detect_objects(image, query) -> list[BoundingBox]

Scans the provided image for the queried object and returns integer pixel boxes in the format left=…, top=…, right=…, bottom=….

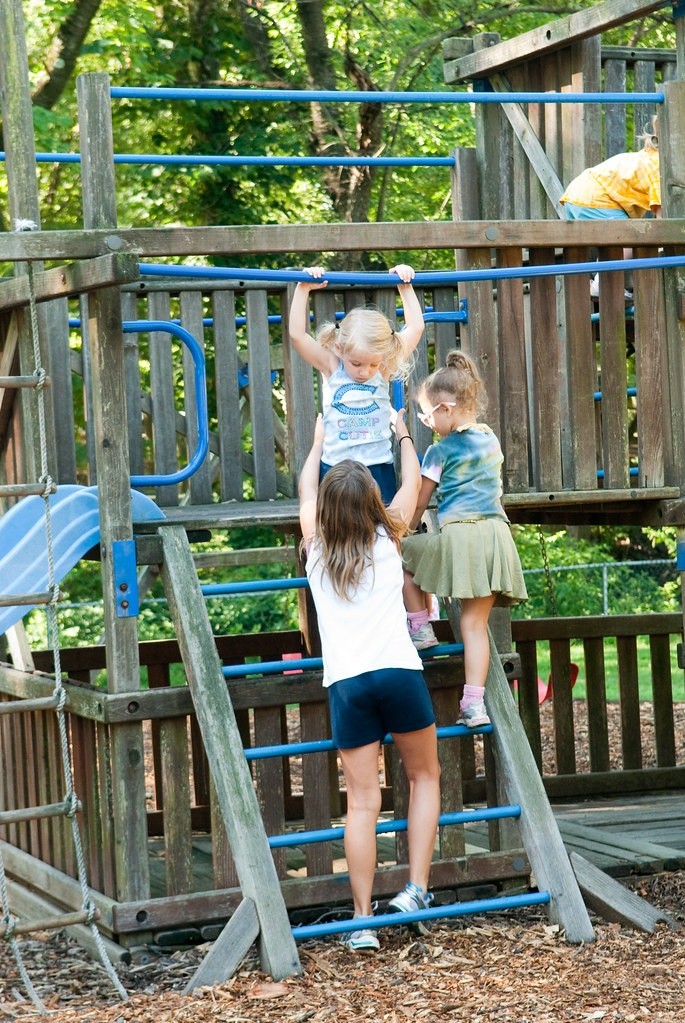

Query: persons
left=401, top=349, right=529, bottom=729
left=559, top=119, right=662, bottom=307
left=288, top=264, right=425, bottom=509
left=297, top=407, right=442, bottom=951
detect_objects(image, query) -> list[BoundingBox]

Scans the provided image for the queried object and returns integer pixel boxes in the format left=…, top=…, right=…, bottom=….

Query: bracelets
left=399, top=436, right=413, bottom=447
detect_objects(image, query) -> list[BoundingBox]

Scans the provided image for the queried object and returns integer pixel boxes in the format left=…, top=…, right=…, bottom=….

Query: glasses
left=417, top=402, right=456, bottom=427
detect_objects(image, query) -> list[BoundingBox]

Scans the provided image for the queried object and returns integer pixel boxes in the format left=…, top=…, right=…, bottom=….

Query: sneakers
left=455, top=700, right=490, bottom=727
left=409, top=623, right=439, bottom=650
left=341, top=913, right=380, bottom=953
left=388, top=882, right=432, bottom=936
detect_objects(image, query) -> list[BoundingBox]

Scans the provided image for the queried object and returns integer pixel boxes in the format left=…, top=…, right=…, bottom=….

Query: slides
left=0, top=482, right=169, bottom=632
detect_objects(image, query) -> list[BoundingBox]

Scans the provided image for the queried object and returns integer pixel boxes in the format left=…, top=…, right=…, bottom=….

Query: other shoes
left=590, top=274, right=633, bottom=298
